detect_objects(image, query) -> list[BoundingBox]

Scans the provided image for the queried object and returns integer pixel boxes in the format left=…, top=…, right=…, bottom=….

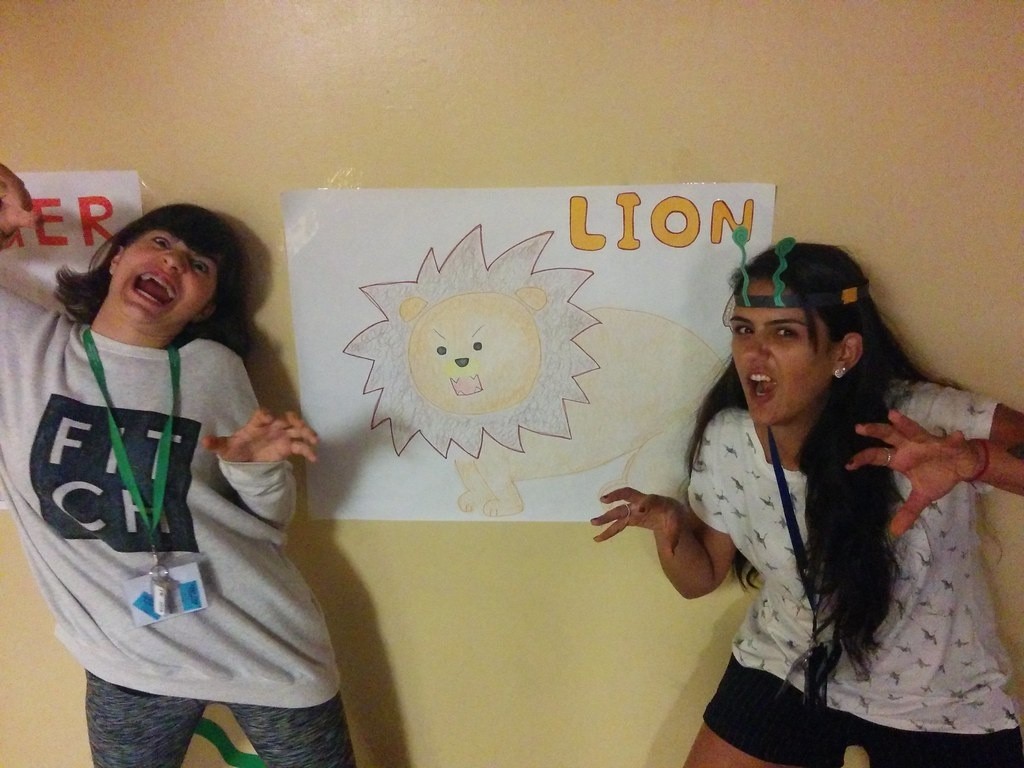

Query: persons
left=590, top=241, right=1023, bottom=768
left=0, top=162, right=355, bottom=768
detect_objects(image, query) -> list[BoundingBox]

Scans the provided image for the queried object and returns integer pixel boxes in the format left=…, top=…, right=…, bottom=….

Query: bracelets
left=964, top=439, right=989, bottom=482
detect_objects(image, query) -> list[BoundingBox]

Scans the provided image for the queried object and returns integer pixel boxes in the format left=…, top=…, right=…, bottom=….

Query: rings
left=625, top=504, right=630, bottom=516
left=884, top=447, right=891, bottom=464
